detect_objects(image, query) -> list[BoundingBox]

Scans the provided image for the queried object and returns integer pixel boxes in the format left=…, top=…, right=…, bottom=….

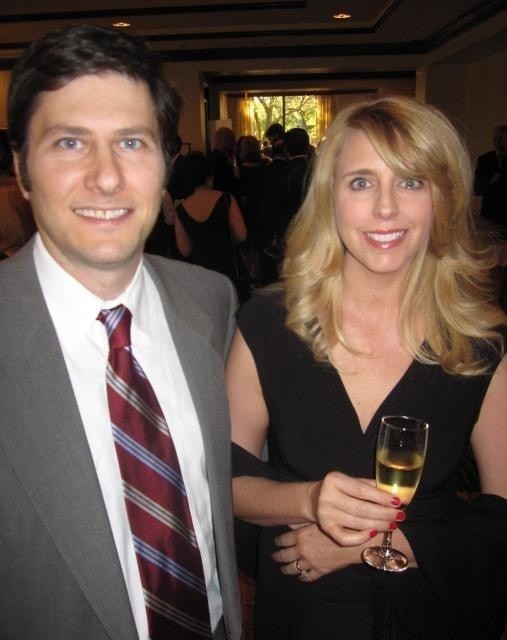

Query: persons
left=146, top=124, right=336, bottom=304
left=221, top=96, right=506, bottom=638
left=1, top=27, right=241, bottom=639
left=473, top=124, right=506, bottom=308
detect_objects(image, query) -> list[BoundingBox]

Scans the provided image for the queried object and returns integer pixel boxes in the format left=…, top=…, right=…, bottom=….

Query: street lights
left=232, top=253, right=248, bottom=280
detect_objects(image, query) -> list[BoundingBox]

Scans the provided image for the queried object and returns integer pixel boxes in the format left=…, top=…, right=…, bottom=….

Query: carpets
left=97, top=305, right=212, bottom=639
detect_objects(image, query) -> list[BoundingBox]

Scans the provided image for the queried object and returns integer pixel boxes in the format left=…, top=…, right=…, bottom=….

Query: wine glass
left=296, top=560, right=302, bottom=573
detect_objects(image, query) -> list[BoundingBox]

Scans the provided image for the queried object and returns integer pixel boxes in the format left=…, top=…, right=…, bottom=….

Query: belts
left=361, top=414, right=431, bottom=572
